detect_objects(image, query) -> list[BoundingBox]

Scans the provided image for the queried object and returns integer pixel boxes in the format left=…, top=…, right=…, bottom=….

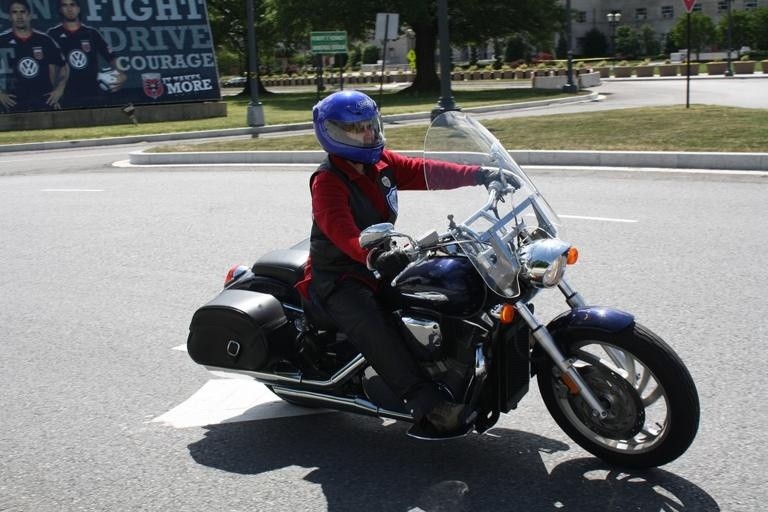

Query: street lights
left=400, top=21, right=416, bottom=49
left=606, top=12, right=621, bottom=57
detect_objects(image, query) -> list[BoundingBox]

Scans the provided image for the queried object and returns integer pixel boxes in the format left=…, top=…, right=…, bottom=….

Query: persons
left=294, top=88, right=523, bottom=434
left=46, top=0, right=127, bottom=110
left=1, top=0, right=70, bottom=111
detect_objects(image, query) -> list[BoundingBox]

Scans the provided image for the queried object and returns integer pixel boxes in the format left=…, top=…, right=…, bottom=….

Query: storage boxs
left=184, top=287, right=291, bottom=371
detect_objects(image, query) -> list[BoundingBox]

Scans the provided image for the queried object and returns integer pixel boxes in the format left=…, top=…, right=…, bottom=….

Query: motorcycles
left=182, top=109, right=700, bottom=472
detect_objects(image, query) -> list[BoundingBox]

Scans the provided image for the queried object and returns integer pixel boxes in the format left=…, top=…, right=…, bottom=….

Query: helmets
left=314, top=91, right=385, bottom=165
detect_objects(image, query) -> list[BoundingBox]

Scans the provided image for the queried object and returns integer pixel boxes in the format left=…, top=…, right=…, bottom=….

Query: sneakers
left=427, top=402, right=477, bottom=428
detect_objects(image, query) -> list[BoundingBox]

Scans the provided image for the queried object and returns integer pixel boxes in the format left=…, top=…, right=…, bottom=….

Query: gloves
left=369, top=250, right=406, bottom=278
left=477, top=168, right=521, bottom=190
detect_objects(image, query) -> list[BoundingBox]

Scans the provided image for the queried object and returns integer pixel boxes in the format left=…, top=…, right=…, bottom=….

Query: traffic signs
left=309, top=30, right=348, bottom=55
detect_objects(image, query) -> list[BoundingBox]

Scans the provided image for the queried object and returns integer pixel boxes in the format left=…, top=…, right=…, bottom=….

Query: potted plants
left=591, top=60, right=610, bottom=78
left=658, top=61, right=678, bottom=76
left=516, top=64, right=532, bottom=79
left=264, top=71, right=392, bottom=88
left=759, top=59, right=768, bottom=73
left=465, top=65, right=480, bottom=80
left=453, top=66, right=464, bottom=80
left=636, top=58, right=655, bottom=77
left=534, top=63, right=550, bottom=77
left=500, top=65, right=515, bottom=80
left=494, top=70, right=502, bottom=80
left=680, top=60, right=699, bottom=74
left=706, top=58, right=729, bottom=75
left=732, top=55, right=757, bottom=75
left=392, top=70, right=407, bottom=82
left=483, top=66, right=492, bottom=79
left=408, top=68, right=416, bottom=82
left=552, top=61, right=567, bottom=76
left=613, top=60, right=634, bottom=78
left=574, top=61, right=589, bottom=73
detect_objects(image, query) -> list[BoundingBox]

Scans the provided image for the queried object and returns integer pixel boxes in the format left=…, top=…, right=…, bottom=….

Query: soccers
left=98, top=67, right=119, bottom=92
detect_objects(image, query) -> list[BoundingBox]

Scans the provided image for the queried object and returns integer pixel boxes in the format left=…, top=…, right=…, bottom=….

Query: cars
left=223, top=77, right=248, bottom=88
